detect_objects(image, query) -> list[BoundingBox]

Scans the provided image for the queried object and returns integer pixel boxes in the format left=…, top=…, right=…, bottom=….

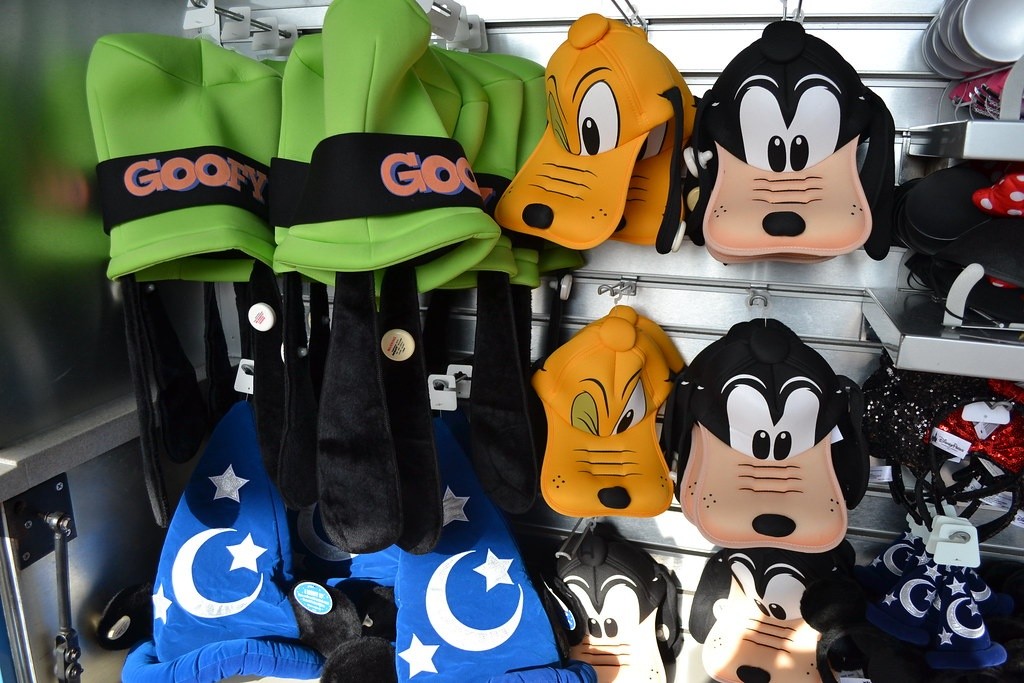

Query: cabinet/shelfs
left=0, top=0, right=1024, bottom=683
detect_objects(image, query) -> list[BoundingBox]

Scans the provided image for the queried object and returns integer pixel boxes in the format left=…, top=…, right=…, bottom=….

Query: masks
left=494, top=13, right=691, bottom=250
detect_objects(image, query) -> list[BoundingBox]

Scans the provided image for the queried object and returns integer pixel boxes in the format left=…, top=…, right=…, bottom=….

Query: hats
left=269, top=0, right=587, bottom=555
left=530, top=307, right=686, bottom=517
left=525, top=521, right=680, bottom=683
left=396, top=422, right=597, bottom=683
left=682, top=21, right=895, bottom=264
left=84, top=34, right=329, bottom=525
left=676, top=318, right=868, bottom=553
left=119, top=400, right=323, bottom=683
left=603, top=25, right=697, bottom=255
left=688, top=538, right=856, bottom=682
